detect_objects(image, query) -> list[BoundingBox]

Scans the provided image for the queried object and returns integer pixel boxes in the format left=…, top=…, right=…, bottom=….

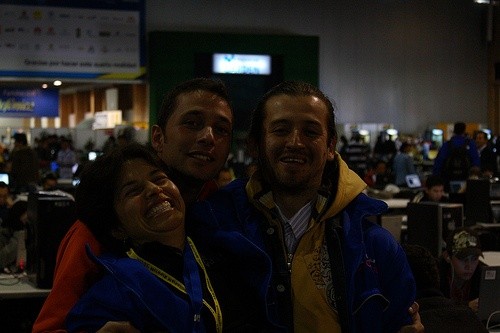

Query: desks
left=0, top=272, right=52, bottom=300
left=358, top=158, right=500, bottom=332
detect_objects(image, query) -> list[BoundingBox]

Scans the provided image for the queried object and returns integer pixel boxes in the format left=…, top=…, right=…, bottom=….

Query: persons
left=0, top=76, right=500, bottom=333
left=63, top=149, right=260, bottom=333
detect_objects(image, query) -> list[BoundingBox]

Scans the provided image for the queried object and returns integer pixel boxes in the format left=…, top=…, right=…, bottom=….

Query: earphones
left=446, top=256, right=450, bottom=262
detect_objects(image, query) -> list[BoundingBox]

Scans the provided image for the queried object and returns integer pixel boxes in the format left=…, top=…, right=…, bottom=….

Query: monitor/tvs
left=476, top=266, right=500, bottom=323
left=404, top=174, right=422, bottom=188
left=0, top=173, right=10, bottom=186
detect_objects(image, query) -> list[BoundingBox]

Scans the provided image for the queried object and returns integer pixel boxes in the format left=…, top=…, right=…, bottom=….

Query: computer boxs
left=407, top=201, right=464, bottom=259
left=25, top=190, right=76, bottom=290
left=448, top=179, right=492, bottom=226
